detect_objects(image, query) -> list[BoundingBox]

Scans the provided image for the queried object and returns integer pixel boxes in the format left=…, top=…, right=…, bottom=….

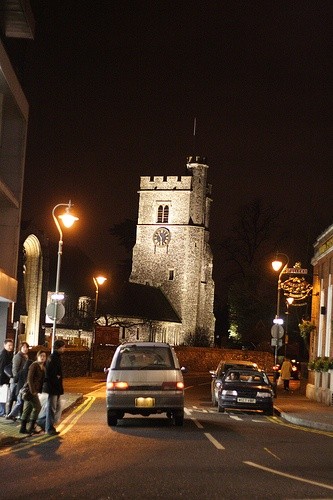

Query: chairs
left=123, top=354, right=136, bottom=365
left=230, top=371, right=240, bottom=380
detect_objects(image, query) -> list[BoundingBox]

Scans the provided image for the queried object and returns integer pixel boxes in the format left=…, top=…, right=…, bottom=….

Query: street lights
left=271, top=252, right=289, bottom=382
left=88, top=272, right=108, bottom=377
left=50, top=200, right=79, bottom=355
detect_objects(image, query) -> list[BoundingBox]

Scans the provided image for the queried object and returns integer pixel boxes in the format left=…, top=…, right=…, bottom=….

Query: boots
left=20, top=421, right=28, bottom=433
left=29, top=421, right=39, bottom=434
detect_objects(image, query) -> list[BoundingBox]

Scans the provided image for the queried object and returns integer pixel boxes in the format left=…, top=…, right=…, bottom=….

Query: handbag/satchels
left=19, top=383, right=34, bottom=401
left=3, top=364, right=14, bottom=377
left=0, top=384, right=12, bottom=403
left=277, top=372, right=283, bottom=386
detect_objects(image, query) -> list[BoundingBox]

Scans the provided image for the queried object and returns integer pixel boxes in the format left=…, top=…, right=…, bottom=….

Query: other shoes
left=37, top=423, right=45, bottom=432
left=5, top=411, right=18, bottom=419
left=11, top=416, right=17, bottom=420
left=11, top=415, right=17, bottom=420
left=46, top=428, right=60, bottom=436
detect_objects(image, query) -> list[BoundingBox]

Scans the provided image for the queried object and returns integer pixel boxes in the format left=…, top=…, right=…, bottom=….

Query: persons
left=20, top=349, right=60, bottom=433
left=281, top=357, right=293, bottom=390
left=0, top=337, right=37, bottom=421
left=227, top=372, right=241, bottom=382
left=43, top=340, right=66, bottom=434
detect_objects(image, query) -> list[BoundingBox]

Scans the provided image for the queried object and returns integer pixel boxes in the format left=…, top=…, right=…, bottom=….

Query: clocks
left=152, top=226, right=172, bottom=248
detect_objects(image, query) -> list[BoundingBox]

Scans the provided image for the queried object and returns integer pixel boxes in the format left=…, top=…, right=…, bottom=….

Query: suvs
left=210, top=359, right=259, bottom=406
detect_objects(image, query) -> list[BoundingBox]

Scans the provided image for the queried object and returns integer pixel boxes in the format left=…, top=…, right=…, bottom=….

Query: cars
left=214, top=368, right=275, bottom=416
left=275, top=360, right=299, bottom=380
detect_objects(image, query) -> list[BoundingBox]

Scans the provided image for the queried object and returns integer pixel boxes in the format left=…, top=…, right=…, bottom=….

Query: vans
left=103, top=341, right=186, bottom=426
